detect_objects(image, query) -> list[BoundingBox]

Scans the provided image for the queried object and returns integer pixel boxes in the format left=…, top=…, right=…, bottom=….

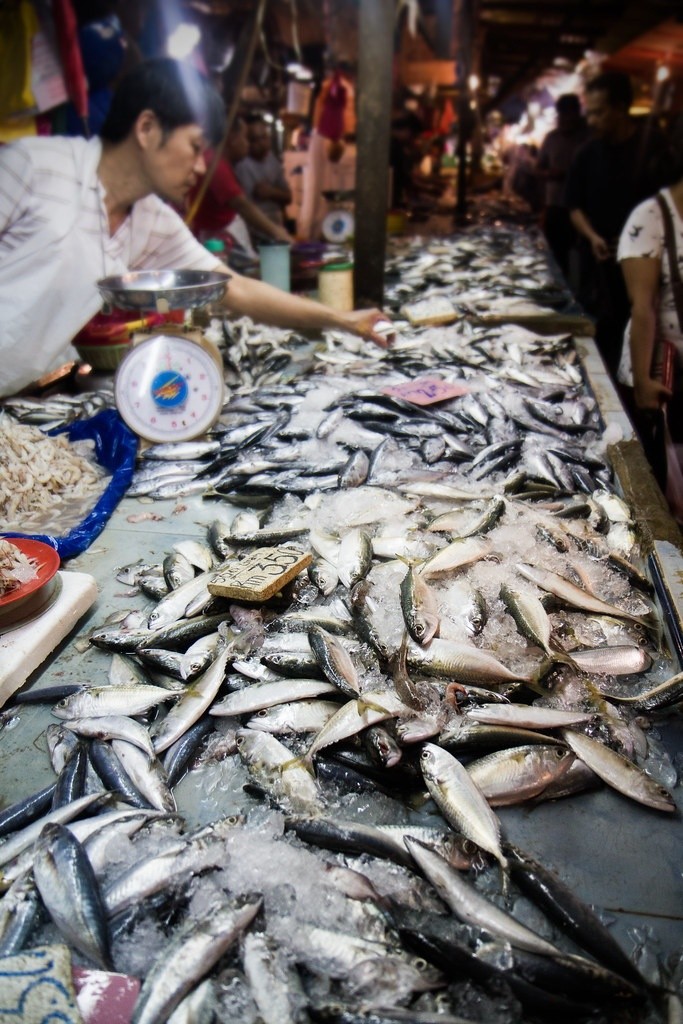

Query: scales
left=320, top=188, right=355, bottom=244
left=92, top=268, right=231, bottom=452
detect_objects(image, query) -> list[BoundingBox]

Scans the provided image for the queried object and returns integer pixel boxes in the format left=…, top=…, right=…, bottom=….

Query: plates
left=0, top=536, right=61, bottom=610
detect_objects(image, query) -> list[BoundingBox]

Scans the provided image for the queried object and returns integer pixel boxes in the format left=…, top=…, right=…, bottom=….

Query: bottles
left=203, top=236, right=228, bottom=267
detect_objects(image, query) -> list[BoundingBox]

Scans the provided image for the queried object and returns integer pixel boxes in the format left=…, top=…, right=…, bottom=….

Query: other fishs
left=5, top=389, right=117, bottom=433
left=122, top=223, right=616, bottom=500
left=0, top=488, right=683, bottom=1024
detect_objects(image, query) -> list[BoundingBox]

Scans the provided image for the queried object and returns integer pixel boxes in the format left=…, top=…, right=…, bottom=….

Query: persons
left=390, top=101, right=425, bottom=211
left=504, top=69, right=664, bottom=370
left=616, top=114, right=683, bottom=492
left=0, top=59, right=395, bottom=400
left=192, top=114, right=296, bottom=251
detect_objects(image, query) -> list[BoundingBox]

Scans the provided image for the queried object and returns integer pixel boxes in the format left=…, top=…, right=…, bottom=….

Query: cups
left=317, top=263, right=354, bottom=313
left=257, top=240, right=291, bottom=295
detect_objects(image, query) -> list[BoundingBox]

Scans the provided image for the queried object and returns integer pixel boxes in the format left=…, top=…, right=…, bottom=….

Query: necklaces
left=97, top=178, right=133, bottom=315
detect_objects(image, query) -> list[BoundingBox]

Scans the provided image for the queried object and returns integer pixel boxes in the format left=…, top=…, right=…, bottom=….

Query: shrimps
left=0, top=410, right=114, bottom=599
